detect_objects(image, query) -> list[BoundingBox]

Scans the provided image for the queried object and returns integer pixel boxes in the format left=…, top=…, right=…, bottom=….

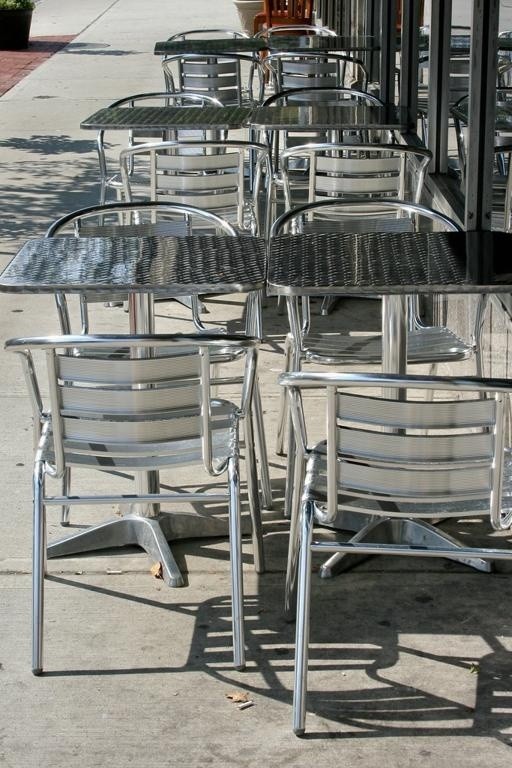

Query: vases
left=235, top=2, right=265, bottom=38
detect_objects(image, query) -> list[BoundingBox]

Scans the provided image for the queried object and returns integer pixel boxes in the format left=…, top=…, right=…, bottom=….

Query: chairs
left=277, top=370, right=512, bottom=738
left=254, top=24, right=336, bottom=60
left=5, top=334, right=263, bottom=675
left=253, top=52, right=370, bottom=95
left=416, top=48, right=473, bottom=144
left=118, top=140, right=273, bottom=228
left=96, top=91, right=228, bottom=226
left=252, top=0, right=313, bottom=83
left=46, top=199, right=241, bottom=334
left=280, top=142, right=434, bottom=231
left=159, top=51, right=267, bottom=194
left=164, top=28, right=258, bottom=102
left=257, top=87, right=384, bottom=240
left=271, top=198, right=465, bottom=375
left=451, top=62, right=511, bottom=198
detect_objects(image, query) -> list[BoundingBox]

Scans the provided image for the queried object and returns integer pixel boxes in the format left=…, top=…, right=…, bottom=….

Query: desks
left=397, top=34, right=512, bottom=53
left=269, top=34, right=378, bottom=60
left=267, top=231, right=512, bottom=580
left=242, top=102, right=403, bottom=318
left=0, top=236, right=267, bottom=589
left=81, top=108, right=246, bottom=314
left=153, top=40, right=267, bottom=57
left=449, top=103, right=511, bottom=132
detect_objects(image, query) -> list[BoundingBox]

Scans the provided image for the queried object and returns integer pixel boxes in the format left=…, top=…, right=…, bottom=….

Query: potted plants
left=0, top=0, right=35, bottom=52
